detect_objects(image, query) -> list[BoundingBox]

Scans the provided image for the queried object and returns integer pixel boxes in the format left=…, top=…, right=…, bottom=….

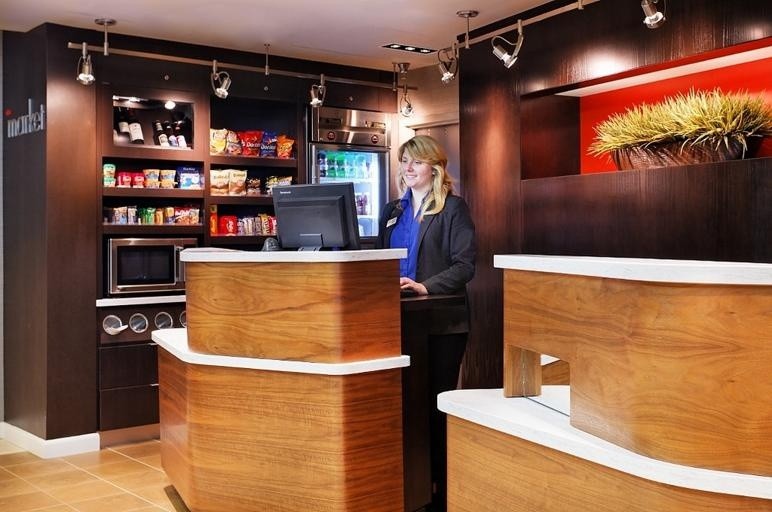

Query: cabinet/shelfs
left=96, top=80, right=307, bottom=447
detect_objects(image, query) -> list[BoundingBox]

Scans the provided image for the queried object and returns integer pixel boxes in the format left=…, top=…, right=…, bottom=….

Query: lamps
left=437, top=0, right=667, bottom=85
left=161, top=99, right=176, bottom=110
left=67, top=41, right=419, bottom=117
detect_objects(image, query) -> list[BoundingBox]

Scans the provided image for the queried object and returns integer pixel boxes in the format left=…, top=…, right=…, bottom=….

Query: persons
left=370, top=135, right=479, bottom=511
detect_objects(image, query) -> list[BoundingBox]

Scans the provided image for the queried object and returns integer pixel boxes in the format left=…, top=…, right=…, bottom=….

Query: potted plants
left=586, top=83, right=772, bottom=171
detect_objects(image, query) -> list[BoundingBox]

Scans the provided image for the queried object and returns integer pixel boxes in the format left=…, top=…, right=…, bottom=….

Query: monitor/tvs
left=270, top=181, right=363, bottom=251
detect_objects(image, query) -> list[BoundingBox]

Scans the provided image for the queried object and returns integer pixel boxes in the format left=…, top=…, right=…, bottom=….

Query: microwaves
left=106, top=238, right=197, bottom=295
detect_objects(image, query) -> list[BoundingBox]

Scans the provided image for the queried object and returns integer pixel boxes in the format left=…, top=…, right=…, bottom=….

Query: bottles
left=114, top=106, right=188, bottom=150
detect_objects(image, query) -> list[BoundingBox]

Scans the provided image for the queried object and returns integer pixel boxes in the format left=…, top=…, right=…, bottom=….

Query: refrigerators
left=306, top=107, right=390, bottom=250
